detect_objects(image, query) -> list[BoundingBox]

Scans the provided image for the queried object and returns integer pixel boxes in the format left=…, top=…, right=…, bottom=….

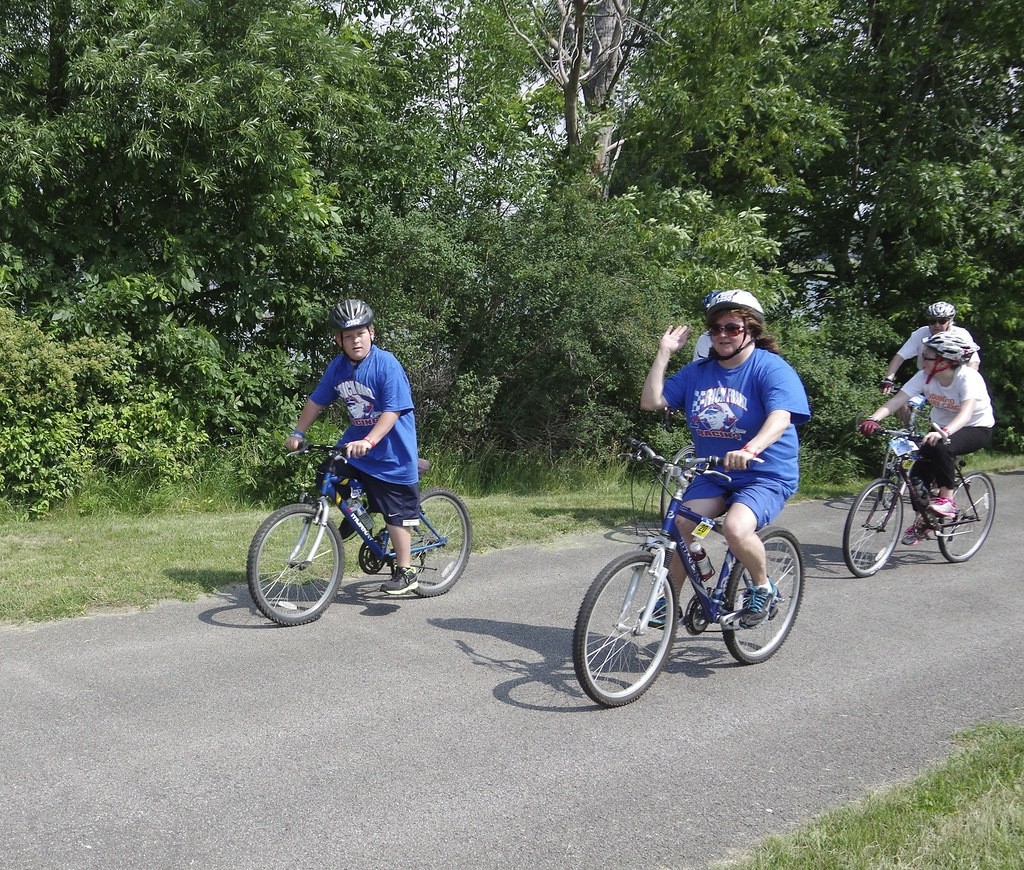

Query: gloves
left=860, top=420, right=881, bottom=436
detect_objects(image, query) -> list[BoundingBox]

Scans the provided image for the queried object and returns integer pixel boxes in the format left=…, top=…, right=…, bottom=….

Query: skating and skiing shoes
left=739, top=577, right=779, bottom=628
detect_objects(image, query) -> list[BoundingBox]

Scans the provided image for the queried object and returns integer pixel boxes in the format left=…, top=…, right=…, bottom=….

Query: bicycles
left=883, top=377, right=933, bottom=509
left=842, top=424, right=996, bottom=577
left=571, top=437, right=806, bottom=707
left=246, top=442, right=473, bottom=626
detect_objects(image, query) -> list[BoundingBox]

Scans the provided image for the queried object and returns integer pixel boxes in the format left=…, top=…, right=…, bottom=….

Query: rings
left=730, top=460, right=735, bottom=463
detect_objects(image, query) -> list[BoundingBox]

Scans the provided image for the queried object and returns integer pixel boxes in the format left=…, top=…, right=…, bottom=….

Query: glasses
left=710, top=323, right=750, bottom=337
left=922, top=353, right=937, bottom=361
left=928, top=318, right=951, bottom=324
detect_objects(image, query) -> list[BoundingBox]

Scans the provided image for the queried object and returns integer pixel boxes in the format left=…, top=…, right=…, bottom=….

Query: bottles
left=347, top=498, right=375, bottom=530
left=911, top=476, right=930, bottom=506
left=930, top=483, right=940, bottom=497
left=689, top=542, right=712, bottom=579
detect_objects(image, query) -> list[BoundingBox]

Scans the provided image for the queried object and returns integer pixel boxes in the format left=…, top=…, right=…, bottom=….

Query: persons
left=879, top=302, right=980, bottom=498
left=692, top=291, right=713, bottom=362
left=284, top=300, right=422, bottom=594
left=857, top=333, right=995, bottom=546
left=640, top=290, right=811, bottom=627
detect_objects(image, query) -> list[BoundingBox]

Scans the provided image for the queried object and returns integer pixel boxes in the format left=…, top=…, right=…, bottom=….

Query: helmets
left=927, top=301, right=956, bottom=318
left=703, top=289, right=764, bottom=323
left=921, top=332, right=981, bottom=360
left=331, top=298, right=374, bottom=329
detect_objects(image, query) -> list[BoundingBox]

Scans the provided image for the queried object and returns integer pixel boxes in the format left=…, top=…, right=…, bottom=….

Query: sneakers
left=380, top=565, right=419, bottom=595
left=335, top=498, right=374, bottom=542
left=927, top=497, right=956, bottom=518
left=639, top=597, right=683, bottom=630
left=901, top=524, right=932, bottom=546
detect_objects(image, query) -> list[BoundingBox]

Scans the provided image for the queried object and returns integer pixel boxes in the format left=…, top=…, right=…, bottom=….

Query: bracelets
left=291, top=431, right=306, bottom=439
left=364, top=437, right=376, bottom=447
left=942, top=427, right=952, bottom=436
left=743, top=446, right=758, bottom=457
